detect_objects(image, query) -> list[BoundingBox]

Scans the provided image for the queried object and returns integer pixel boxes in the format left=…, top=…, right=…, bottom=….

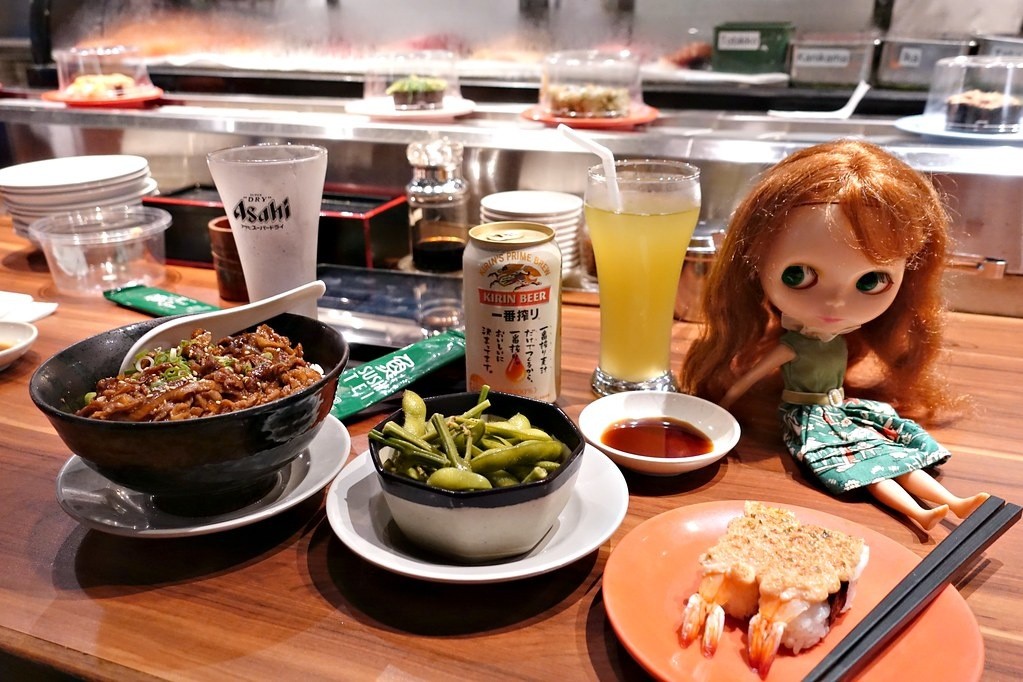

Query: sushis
left=679, top=503, right=871, bottom=674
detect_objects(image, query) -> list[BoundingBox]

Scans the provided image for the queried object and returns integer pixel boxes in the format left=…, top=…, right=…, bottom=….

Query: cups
left=208, top=215, right=250, bottom=304
left=206, top=142, right=328, bottom=320
left=586, top=158, right=703, bottom=399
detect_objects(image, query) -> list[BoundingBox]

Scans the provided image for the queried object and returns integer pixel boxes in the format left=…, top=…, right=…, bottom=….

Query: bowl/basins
left=27, top=310, right=348, bottom=518
left=368, top=391, right=585, bottom=561
left=1, top=154, right=174, bottom=295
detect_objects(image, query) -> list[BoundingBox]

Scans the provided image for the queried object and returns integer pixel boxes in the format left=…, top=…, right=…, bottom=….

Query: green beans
left=369, top=383, right=571, bottom=492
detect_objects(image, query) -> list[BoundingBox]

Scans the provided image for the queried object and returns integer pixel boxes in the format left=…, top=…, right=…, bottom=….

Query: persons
left=681, top=137, right=992, bottom=533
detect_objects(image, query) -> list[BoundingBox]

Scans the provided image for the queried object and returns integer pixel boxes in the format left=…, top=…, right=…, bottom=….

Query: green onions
left=84, top=339, right=273, bottom=405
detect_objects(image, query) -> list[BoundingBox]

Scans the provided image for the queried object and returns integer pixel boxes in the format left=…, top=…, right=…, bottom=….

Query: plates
left=603, top=499, right=987, bottom=682
left=326, top=441, right=633, bottom=584
left=0, top=320, right=38, bottom=371
left=520, top=103, right=659, bottom=134
left=57, top=414, right=352, bottom=538
left=894, top=112, right=1023, bottom=145
left=40, top=85, right=164, bottom=108
left=480, top=190, right=585, bottom=281
left=577, top=389, right=741, bottom=477
left=344, top=96, right=475, bottom=122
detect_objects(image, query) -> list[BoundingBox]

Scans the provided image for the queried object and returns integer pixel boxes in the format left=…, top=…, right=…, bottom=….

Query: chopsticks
left=800, top=494, right=1023, bottom=682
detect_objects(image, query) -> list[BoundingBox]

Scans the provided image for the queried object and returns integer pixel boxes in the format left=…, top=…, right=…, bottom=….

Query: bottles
left=674, top=219, right=733, bottom=324
left=407, top=139, right=471, bottom=273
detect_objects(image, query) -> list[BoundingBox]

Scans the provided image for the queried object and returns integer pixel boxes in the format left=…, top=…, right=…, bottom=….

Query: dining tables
left=0, top=206, right=1023, bottom=681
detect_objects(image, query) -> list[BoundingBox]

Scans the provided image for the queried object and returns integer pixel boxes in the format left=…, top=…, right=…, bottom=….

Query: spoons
left=118, top=280, right=326, bottom=377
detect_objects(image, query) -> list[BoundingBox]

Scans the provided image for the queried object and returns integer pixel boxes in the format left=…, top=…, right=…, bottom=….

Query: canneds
left=461, top=219, right=564, bottom=404
